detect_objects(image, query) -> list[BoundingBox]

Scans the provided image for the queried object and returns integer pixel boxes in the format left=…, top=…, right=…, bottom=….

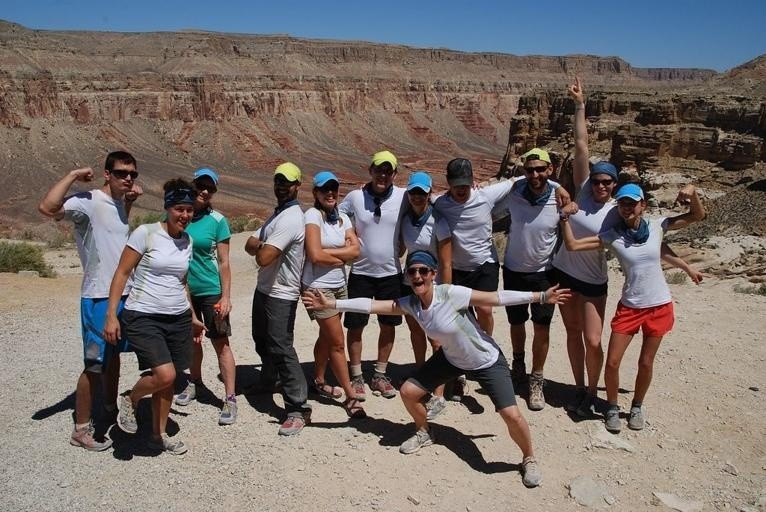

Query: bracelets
left=124, top=192, right=137, bottom=202
left=573, top=103, right=584, bottom=110
left=256, top=242, right=263, bottom=249
left=559, top=213, right=570, bottom=223
left=538, top=291, right=546, bottom=305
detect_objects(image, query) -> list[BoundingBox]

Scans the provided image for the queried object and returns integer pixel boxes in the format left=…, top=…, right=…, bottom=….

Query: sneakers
left=521, top=456, right=539, bottom=488
left=399, top=425, right=435, bottom=454
left=69, top=376, right=238, bottom=455
left=242, top=368, right=398, bottom=436
left=566, top=387, right=644, bottom=431
left=425, top=359, right=545, bottom=420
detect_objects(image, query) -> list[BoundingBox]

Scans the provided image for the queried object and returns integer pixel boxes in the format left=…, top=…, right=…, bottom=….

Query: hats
left=616, top=183, right=644, bottom=201
left=521, top=148, right=552, bottom=164
left=313, top=170, right=339, bottom=188
left=407, top=172, right=432, bottom=194
left=274, top=161, right=302, bottom=183
left=193, top=168, right=218, bottom=185
left=590, top=161, right=618, bottom=182
left=371, top=150, right=398, bottom=171
left=447, top=158, right=472, bottom=187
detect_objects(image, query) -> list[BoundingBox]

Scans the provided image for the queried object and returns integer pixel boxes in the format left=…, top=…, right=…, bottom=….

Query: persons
left=397, top=171, right=453, bottom=420
left=302, top=249, right=573, bottom=486
left=336, top=151, right=484, bottom=402
left=160, top=168, right=237, bottom=426
left=102, top=179, right=209, bottom=456
left=303, top=170, right=366, bottom=420
left=244, top=161, right=314, bottom=437
left=491, top=147, right=564, bottom=412
left=551, top=77, right=712, bottom=417
left=38, top=150, right=144, bottom=451
left=359, top=157, right=573, bottom=403
left=558, top=182, right=704, bottom=433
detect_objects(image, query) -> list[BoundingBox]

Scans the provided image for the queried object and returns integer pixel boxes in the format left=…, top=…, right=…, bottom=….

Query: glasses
left=174, top=189, right=198, bottom=200
left=407, top=267, right=432, bottom=275
left=374, top=197, right=381, bottom=216
left=592, top=179, right=612, bottom=185
left=524, top=166, right=549, bottom=172
left=111, top=170, right=138, bottom=179
left=195, top=183, right=217, bottom=193
